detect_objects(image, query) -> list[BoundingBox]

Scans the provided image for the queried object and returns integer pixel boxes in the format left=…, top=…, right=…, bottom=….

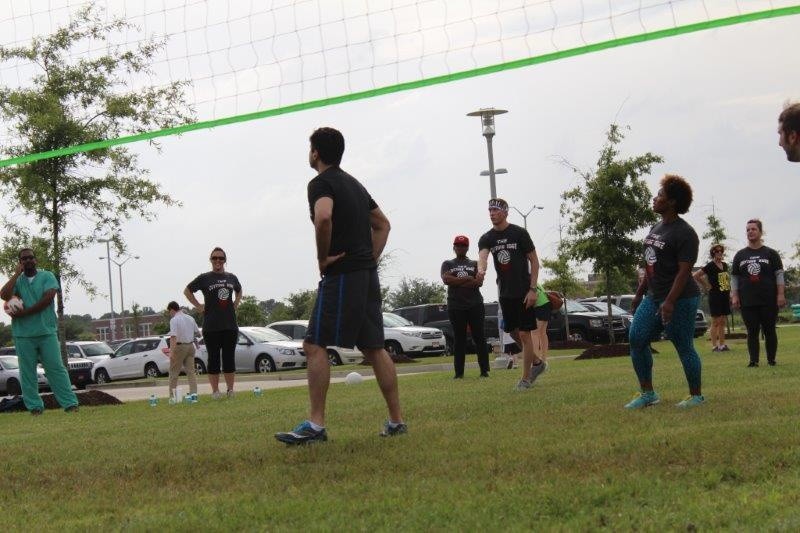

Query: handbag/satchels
left=546, top=291, right=563, bottom=310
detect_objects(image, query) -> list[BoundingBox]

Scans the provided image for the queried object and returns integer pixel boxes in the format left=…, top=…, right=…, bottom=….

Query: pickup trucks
left=391, top=303, right=500, bottom=354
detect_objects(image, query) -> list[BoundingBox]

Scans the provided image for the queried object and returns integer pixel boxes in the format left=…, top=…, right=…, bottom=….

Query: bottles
left=191, top=392, right=198, bottom=403
left=185, top=392, right=191, bottom=403
left=149, top=395, right=157, bottom=407
left=254, top=387, right=259, bottom=395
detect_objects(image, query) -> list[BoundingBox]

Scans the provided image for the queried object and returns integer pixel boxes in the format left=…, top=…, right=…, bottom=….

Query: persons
left=275, top=127, right=407, bottom=444
left=0, top=248, right=79, bottom=414
left=439, top=198, right=552, bottom=389
left=692, top=244, right=734, bottom=352
left=167, top=302, right=201, bottom=399
left=625, top=174, right=707, bottom=410
left=184, top=248, right=242, bottom=398
left=730, top=220, right=784, bottom=367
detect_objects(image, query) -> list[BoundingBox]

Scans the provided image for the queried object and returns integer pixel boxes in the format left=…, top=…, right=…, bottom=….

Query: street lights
left=509, top=205, right=544, bottom=230
left=465, top=104, right=509, bottom=199
left=98, top=239, right=119, bottom=340
left=99, top=254, right=142, bottom=338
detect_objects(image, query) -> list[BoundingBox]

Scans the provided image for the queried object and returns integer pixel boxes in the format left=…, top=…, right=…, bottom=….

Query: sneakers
left=676, top=394, right=704, bottom=405
left=380, top=422, right=408, bottom=436
left=274, top=421, right=328, bottom=444
left=626, top=391, right=660, bottom=408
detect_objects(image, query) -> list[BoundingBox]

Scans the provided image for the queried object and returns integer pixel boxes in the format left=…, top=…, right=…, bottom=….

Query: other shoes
left=213, top=390, right=221, bottom=399
left=31, top=407, right=43, bottom=415
left=713, top=345, right=730, bottom=351
left=227, top=389, right=235, bottom=397
left=64, top=406, right=78, bottom=413
left=767, top=360, right=776, bottom=367
left=515, top=360, right=546, bottom=389
left=748, top=362, right=758, bottom=367
left=507, top=358, right=518, bottom=369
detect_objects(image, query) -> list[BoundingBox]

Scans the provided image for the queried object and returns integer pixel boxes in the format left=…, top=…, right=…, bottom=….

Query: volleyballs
left=345, top=371, right=364, bottom=384
left=3, top=296, right=25, bottom=315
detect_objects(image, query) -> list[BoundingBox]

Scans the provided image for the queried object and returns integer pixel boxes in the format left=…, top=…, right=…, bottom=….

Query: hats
left=454, top=236, right=469, bottom=247
left=710, top=245, right=724, bottom=257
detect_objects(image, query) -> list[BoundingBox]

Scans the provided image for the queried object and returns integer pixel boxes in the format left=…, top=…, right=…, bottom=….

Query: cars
left=59, top=340, right=116, bottom=365
left=90, top=334, right=186, bottom=384
left=381, top=312, right=447, bottom=361
left=500, top=294, right=709, bottom=349
left=0, top=345, right=94, bottom=397
left=265, top=320, right=367, bottom=368
left=790, top=304, right=800, bottom=323
left=196, top=326, right=308, bottom=374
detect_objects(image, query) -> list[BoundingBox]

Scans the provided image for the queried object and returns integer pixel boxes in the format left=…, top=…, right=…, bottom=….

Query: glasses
left=211, top=256, right=226, bottom=261
left=712, top=249, right=724, bottom=253
left=20, top=254, right=34, bottom=262
